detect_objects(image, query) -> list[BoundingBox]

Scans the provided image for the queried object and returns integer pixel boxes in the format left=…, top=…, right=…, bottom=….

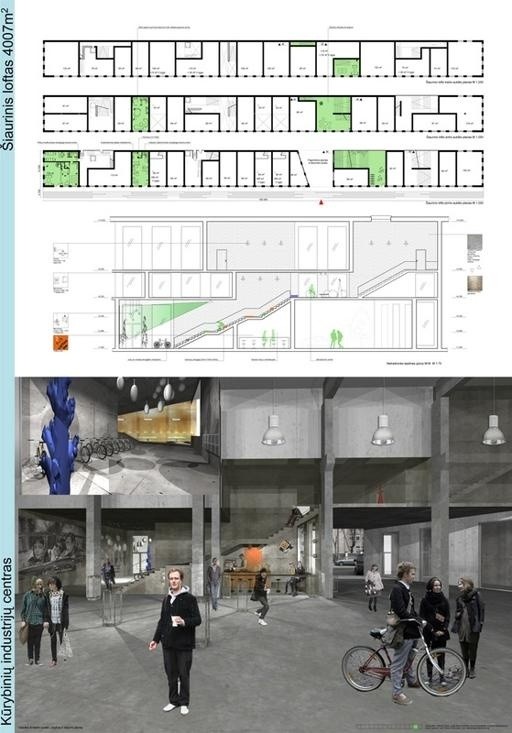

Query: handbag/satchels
left=18, top=621, right=31, bottom=644
left=250, top=589, right=260, bottom=601
left=382, top=609, right=407, bottom=650
left=450, top=616, right=461, bottom=634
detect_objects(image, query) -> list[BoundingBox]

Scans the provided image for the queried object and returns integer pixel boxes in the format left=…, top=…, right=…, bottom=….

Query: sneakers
left=34, top=658, right=45, bottom=667
left=390, top=690, right=414, bottom=707
left=179, top=703, right=191, bottom=715
left=468, top=667, right=477, bottom=678
left=464, top=666, right=470, bottom=678
left=24, top=658, right=35, bottom=666
left=162, top=700, right=180, bottom=712
left=438, top=674, right=449, bottom=687
left=423, top=674, right=433, bottom=687
left=257, top=617, right=268, bottom=626
left=48, top=659, right=59, bottom=668
left=254, top=610, right=263, bottom=616
left=407, top=676, right=425, bottom=689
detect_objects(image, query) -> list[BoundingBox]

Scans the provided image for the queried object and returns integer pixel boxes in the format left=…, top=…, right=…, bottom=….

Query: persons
left=208, top=558, right=221, bottom=611
left=26, top=533, right=77, bottom=567
left=289, top=562, right=306, bottom=596
left=109, top=561, right=116, bottom=585
left=365, top=564, right=385, bottom=611
left=21, top=576, right=46, bottom=666
left=451, top=576, right=485, bottom=678
left=150, top=569, right=202, bottom=715
left=389, top=562, right=420, bottom=705
left=44, top=575, right=69, bottom=668
left=101, top=564, right=111, bottom=590
left=236, top=553, right=247, bottom=571
left=420, top=576, right=448, bottom=687
left=254, top=567, right=269, bottom=626
